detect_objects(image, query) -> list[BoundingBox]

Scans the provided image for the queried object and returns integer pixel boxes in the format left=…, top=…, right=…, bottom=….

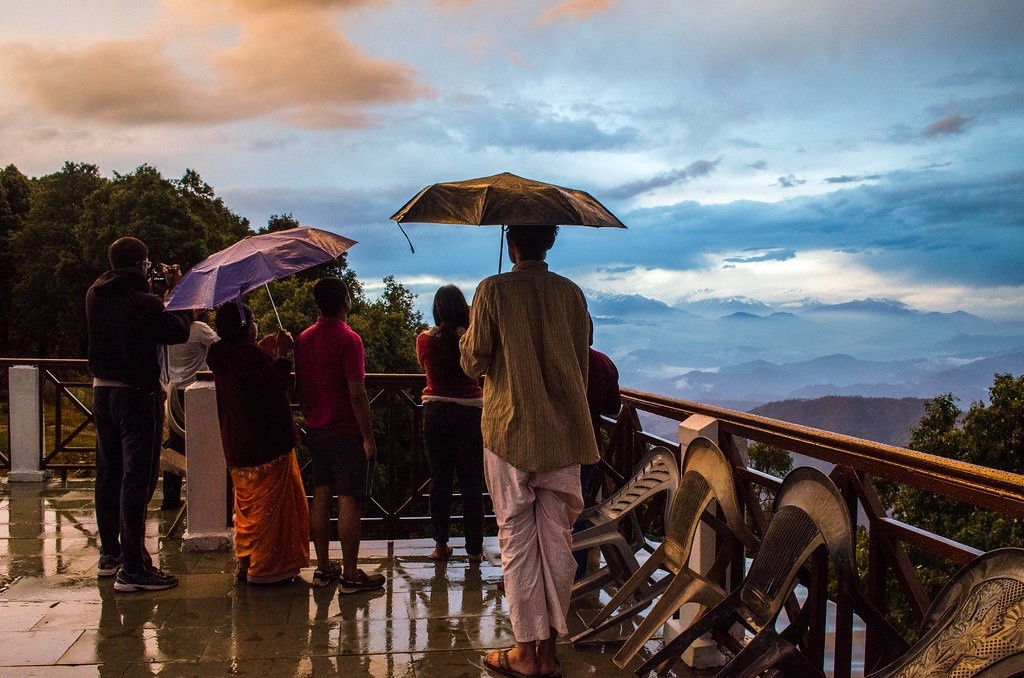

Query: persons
left=416, top=284, right=489, bottom=563
left=85, top=237, right=178, bottom=591
left=205, top=303, right=310, bottom=588
left=159, top=309, right=221, bottom=511
left=294, top=278, right=386, bottom=593
left=568, top=312, right=621, bottom=582
left=459, top=224, right=600, bottom=678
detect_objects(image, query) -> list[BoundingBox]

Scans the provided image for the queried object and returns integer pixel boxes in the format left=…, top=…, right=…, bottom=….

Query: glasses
left=250, top=319, right=260, bottom=326
left=136, top=261, right=152, bottom=268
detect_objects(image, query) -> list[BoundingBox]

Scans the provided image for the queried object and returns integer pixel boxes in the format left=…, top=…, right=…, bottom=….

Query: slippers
left=483, top=651, right=537, bottom=678
left=539, top=654, right=562, bottom=678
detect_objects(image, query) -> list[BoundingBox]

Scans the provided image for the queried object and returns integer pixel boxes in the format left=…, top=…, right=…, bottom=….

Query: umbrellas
left=391, top=172, right=628, bottom=275
left=164, top=225, right=359, bottom=330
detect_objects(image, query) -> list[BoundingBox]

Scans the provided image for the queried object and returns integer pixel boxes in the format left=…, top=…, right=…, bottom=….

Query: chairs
left=572, top=436, right=1024, bottom=678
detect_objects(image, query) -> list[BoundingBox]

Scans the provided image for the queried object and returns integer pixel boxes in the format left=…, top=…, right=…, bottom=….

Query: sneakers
left=96, top=552, right=125, bottom=576
left=313, top=564, right=342, bottom=588
left=338, top=567, right=387, bottom=593
left=114, top=567, right=179, bottom=591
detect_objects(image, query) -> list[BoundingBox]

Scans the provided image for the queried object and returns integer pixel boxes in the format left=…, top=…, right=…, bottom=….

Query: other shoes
left=432, top=545, right=452, bottom=559
left=468, top=552, right=482, bottom=562
left=161, top=501, right=184, bottom=510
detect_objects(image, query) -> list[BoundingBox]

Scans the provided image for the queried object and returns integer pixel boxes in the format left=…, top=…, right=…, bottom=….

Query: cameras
left=152, top=264, right=175, bottom=285
